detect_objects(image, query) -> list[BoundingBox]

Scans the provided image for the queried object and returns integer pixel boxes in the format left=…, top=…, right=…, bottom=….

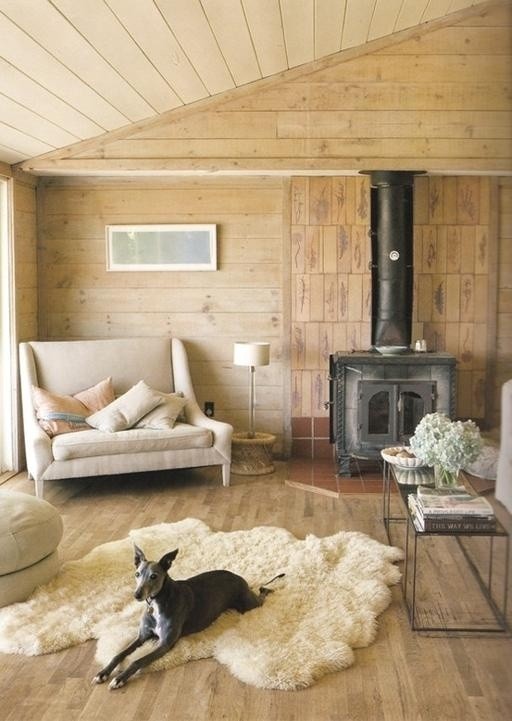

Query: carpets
left=0, top=518, right=405, bottom=691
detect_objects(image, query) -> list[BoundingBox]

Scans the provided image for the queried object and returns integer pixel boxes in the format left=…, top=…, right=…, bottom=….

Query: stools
left=0, top=489, right=64, bottom=610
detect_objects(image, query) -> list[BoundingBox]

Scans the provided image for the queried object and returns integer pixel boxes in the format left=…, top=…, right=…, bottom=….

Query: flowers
left=409, top=413, right=485, bottom=484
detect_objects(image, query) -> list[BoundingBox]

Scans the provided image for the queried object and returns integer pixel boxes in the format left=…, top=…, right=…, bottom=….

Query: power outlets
left=205, top=402, right=214, bottom=417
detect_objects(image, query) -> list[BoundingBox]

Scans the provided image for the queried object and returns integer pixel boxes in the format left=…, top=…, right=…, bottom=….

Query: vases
left=433, top=458, right=461, bottom=490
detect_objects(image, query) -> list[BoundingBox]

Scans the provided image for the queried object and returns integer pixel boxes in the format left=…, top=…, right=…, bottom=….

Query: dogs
left=90, top=543, right=286, bottom=692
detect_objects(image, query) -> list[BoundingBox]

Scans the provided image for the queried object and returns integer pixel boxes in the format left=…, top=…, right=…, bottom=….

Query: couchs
left=19, top=337, right=233, bottom=499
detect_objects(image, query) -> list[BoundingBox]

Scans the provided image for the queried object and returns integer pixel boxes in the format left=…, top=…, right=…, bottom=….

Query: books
left=405, top=486, right=498, bottom=535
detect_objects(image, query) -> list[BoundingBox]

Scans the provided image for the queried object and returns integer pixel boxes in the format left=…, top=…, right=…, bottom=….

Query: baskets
left=393, top=468, right=436, bottom=485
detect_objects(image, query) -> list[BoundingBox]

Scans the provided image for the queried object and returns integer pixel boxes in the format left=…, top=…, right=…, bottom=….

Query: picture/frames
left=104, top=224, right=218, bottom=272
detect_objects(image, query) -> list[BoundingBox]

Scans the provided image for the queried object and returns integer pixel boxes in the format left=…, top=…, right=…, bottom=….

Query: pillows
left=114, top=391, right=186, bottom=423
left=134, top=390, right=188, bottom=432
left=75, top=376, right=115, bottom=414
left=85, top=380, right=165, bottom=434
left=30, top=385, right=96, bottom=437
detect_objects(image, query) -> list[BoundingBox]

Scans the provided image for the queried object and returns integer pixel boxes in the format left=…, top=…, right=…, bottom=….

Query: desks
left=384, top=458, right=509, bottom=632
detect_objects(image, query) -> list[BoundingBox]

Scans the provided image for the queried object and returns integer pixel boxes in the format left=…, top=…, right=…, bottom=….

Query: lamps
left=230, top=341, right=276, bottom=476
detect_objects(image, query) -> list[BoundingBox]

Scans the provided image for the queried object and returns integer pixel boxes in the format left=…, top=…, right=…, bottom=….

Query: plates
left=375, top=346, right=408, bottom=356
left=394, top=470, right=436, bottom=486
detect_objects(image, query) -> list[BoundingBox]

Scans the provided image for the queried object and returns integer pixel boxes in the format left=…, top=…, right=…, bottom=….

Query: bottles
left=415, top=339, right=427, bottom=352
